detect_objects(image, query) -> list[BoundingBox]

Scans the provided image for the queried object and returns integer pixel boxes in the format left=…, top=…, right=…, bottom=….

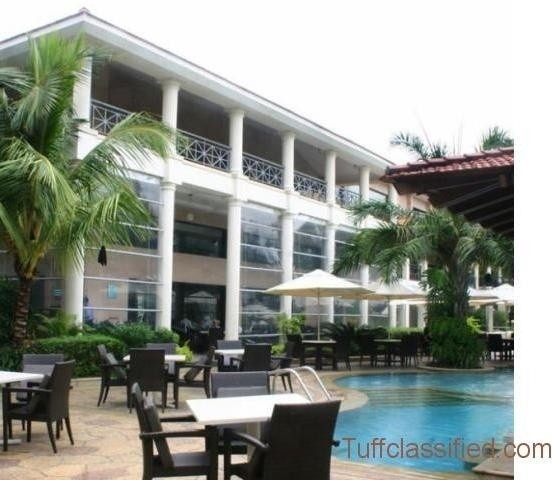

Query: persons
left=82, top=296, right=95, bottom=327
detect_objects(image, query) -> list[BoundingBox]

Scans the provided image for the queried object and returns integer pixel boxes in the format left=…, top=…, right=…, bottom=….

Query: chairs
left=488, top=334, right=512, bottom=362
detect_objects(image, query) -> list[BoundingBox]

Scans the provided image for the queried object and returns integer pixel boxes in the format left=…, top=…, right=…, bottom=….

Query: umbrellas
left=491, top=283, right=514, bottom=339
left=391, top=284, right=499, bottom=304
left=338, top=275, right=440, bottom=340
left=261, top=267, right=375, bottom=341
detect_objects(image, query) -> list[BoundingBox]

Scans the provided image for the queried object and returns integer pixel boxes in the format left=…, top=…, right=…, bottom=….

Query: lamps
left=187, top=193, right=194, bottom=221
